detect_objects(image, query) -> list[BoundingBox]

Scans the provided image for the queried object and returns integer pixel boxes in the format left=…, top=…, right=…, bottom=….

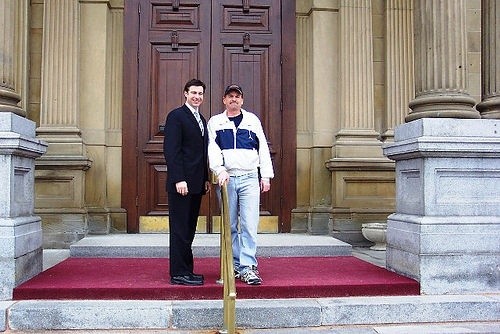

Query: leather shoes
left=170, top=273, right=204, bottom=286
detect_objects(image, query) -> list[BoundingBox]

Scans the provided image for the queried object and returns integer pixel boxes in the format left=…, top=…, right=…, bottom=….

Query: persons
left=207, top=84, right=275, bottom=285
left=163, top=78, right=211, bottom=286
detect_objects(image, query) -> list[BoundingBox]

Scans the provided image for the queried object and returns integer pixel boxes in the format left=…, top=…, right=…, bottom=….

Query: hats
left=225, top=84, right=244, bottom=97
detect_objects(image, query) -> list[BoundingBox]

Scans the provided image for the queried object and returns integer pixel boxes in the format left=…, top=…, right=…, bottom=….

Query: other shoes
left=235, top=264, right=262, bottom=285
